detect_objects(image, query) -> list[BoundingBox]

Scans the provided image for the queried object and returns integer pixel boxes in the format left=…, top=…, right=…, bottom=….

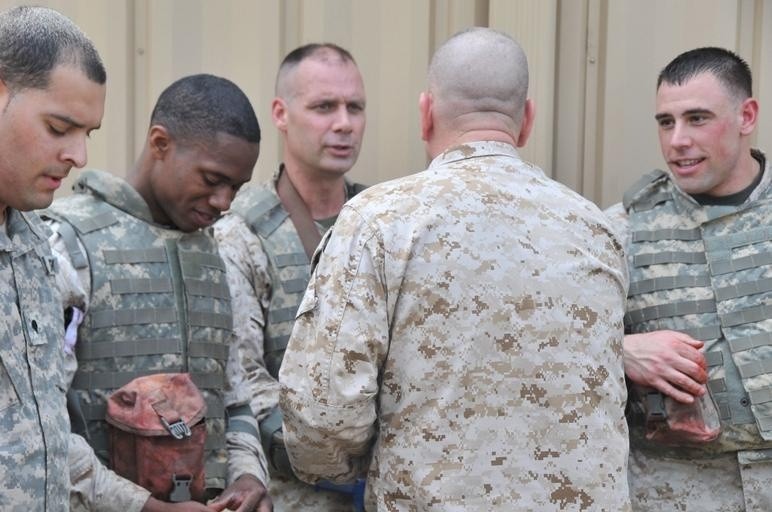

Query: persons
left=278, top=26, right=632, bottom=511
left=603, top=47, right=768, bottom=511
left=230, top=42, right=369, bottom=512
left=38, top=72, right=275, bottom=510
left=1, top=5, right=108, bottom=512
